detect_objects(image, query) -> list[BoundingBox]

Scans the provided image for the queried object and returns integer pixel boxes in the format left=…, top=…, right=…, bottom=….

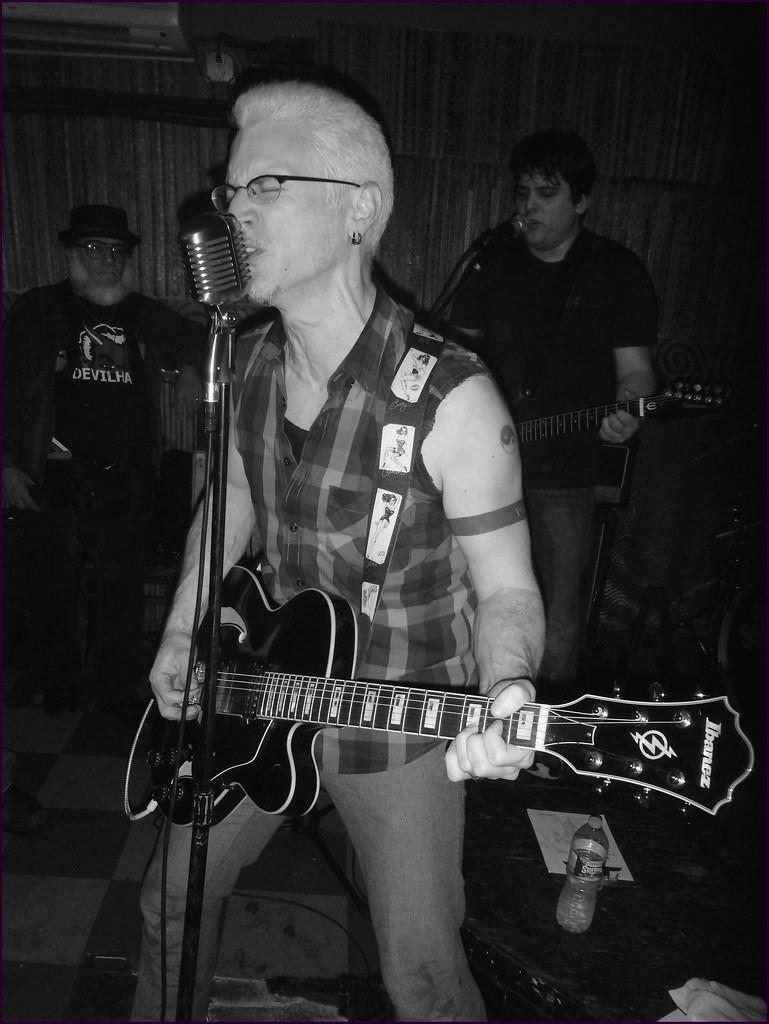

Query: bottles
left=556, top=816, right=610, bottom=933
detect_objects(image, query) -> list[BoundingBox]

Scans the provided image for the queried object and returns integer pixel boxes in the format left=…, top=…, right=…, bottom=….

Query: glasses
left=209, top=174, right=359, bottom=215
left=72, top=241, right=127, bottom=261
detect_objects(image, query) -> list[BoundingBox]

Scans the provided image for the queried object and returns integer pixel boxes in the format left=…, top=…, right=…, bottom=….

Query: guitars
left=122, top=560, right=755, bottom=828
left=513, top=377, right=735, bottom=472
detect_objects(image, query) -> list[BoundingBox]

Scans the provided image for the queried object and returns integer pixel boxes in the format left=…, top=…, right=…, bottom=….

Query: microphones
left=176, top=210, right=252, bottom=433
left=481, top=213, right=531, bottom=250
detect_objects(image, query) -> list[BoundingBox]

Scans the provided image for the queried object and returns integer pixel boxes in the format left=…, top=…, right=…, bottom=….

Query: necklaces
left=74, top=294, right=116, bottom=331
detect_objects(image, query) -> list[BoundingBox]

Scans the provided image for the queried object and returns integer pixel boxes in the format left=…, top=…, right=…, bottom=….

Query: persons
left=445, top=127, right=662, bottom=712
left=133, top=78, right=546, bottom=1023
left=0, top=205, right=209, bottom=733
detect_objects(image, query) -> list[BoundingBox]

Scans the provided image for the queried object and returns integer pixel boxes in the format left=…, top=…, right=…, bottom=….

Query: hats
left=57, top=204, right=141, bottom=249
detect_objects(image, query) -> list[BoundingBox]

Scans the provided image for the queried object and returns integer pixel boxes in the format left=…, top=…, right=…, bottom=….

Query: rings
left=471, top=776, right=482, bottom=781
